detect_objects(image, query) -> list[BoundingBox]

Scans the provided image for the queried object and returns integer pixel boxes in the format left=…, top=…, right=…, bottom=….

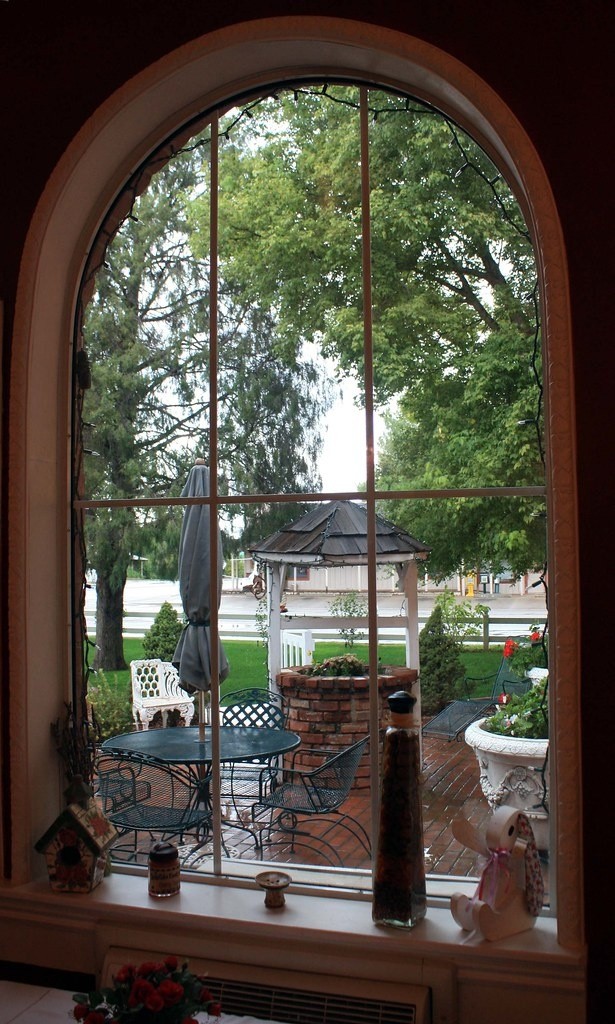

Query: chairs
left=88, top=745, right=229, bottom=871
left=250, top=728, right=385, bottom=869
left=129, top=658, right=195, bottom=730
left=90, top=719, right=156, bottom=844
left=200, top=687, right=290, bottom=847
left=422, top=632, right=539, bottom=744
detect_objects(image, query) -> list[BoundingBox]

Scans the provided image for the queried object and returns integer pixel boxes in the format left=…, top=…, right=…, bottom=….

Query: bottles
left=372, top=691, right=427, bottom=927
left=149, top=842, right=181, bottom=898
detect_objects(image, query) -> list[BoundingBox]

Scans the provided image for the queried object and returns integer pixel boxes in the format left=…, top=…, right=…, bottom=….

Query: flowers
left=303, top=649, right=368, bottom=677
left=502, top=618, right=550, bottom=678
left=68, top=958, right=220, bottom=1024
left=481, top=672, right=548, bottom=740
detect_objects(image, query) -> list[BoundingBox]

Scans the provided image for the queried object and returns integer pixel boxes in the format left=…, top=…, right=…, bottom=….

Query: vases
left=522, top=667, right=550, bottom=689
left=463, top=716, right=550, bottom=907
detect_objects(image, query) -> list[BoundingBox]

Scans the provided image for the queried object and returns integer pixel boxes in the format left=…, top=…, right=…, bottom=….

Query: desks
left=101, top=725, right=302, bottom=859
left=0, top=979, right=288, bottom=1024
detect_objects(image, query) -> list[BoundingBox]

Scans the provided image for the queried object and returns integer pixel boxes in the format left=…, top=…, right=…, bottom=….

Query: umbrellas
left=172, top=458, right=228, bottom=780
